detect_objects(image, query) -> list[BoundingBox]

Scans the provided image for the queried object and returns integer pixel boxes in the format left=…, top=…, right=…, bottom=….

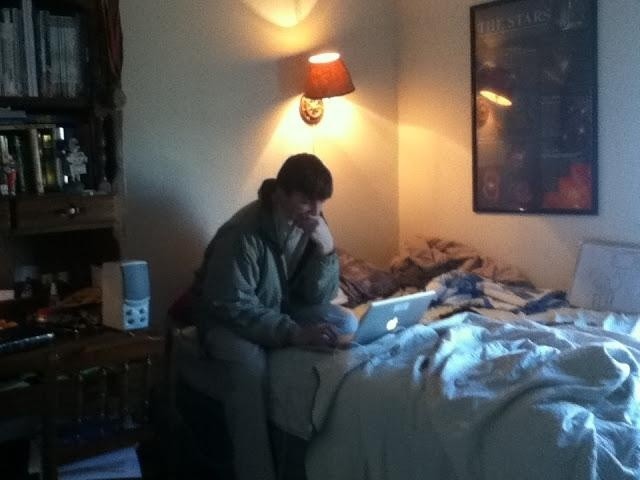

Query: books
left=0, top=106, right=67, bottom=196
left=0, top=1, right=92, bottom=101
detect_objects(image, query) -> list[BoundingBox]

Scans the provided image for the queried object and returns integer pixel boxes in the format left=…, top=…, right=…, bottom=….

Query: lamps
left=300, top=53, right=353, bottom=126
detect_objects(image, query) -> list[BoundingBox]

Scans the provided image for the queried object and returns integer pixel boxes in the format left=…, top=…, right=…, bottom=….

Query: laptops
left=320, top=289, right=437, bottom=349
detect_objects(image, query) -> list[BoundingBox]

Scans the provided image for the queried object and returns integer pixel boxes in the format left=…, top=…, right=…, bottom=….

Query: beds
left=167, top=258, right=639, bottom=478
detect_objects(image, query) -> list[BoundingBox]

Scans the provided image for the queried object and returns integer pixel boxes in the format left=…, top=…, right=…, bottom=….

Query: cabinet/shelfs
left=1, top=2, right=126, bottom=323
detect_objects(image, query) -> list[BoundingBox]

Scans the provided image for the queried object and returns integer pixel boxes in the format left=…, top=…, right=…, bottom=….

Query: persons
left=183, top=152, right=361, bottom=479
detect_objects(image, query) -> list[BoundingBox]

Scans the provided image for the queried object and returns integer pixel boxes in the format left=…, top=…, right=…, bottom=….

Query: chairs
left=44, top=328, right=179, bottom=479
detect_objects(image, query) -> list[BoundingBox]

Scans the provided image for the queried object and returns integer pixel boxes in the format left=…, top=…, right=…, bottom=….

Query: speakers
left=98, top=258, right=152, bottom=331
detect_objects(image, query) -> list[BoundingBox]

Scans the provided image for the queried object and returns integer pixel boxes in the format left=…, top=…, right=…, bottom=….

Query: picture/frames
left=469, top=2, right=600, bottom=216
